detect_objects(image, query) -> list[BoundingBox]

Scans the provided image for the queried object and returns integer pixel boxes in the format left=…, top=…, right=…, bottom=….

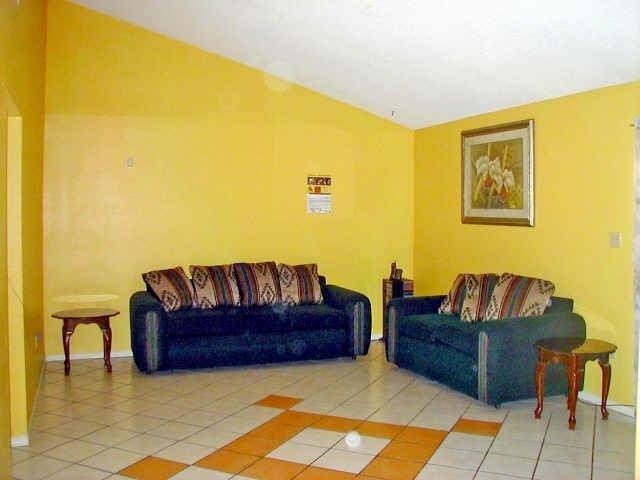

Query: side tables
left=51, top=307, right=120, bottom=376
left=535, top=337, right=618, bottom=429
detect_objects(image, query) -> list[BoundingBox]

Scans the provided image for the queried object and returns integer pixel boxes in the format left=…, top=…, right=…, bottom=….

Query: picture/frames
left=460, top=119, right=535, bottom=227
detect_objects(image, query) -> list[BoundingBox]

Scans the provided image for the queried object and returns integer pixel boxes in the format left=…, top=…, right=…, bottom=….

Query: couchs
left=129, top=285, right=371, bottom=373
left=386, top=295, right=586, bottom=409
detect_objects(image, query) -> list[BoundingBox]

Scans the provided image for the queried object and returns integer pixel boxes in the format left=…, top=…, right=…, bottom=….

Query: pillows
left=460, top=273, right=500, bottom=323
left=234, top=262, right=282, bottom=307
left=277, top=263, right=325, bottom=305
left=438, top=273, right=466, bottom=315
left=482, top=272, right=555, bottom=322
left=142, top=266, right=199, bottom=312
left=190, top=264, right=241, bottom=309
